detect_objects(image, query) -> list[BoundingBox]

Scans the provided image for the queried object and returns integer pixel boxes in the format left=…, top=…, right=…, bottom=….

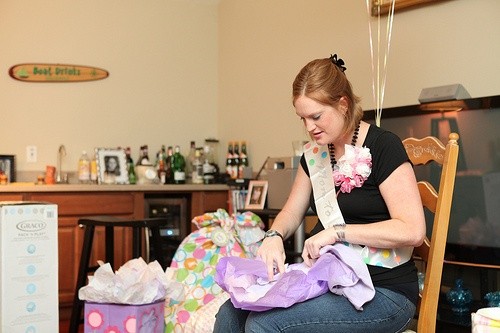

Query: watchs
left=263, top=230, right=283, bottom=238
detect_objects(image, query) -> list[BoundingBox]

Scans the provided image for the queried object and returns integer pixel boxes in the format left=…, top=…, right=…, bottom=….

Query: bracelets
left=332, top=224, right=347, bottom=243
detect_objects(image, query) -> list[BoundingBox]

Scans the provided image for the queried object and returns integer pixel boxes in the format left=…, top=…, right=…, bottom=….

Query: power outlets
left=26, top=145, right=37, bottom=163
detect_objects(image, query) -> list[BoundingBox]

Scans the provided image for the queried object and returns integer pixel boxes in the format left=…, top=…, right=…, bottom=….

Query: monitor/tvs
left=361, top=95, right=500, bottom=259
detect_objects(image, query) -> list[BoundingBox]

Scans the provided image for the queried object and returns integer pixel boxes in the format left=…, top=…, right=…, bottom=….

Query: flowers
left=332, top=144, right=376, bottom=196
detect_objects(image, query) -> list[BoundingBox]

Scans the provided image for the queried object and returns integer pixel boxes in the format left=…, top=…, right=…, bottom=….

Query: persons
left=211, top=54, right=426, bottom=332
left=104, top=157, right=121, bottom=176
left=249, top=187, right=263, bottom=204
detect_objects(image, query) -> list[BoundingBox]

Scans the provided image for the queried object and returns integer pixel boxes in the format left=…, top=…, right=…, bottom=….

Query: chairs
left=402, top=133, right=459, bottom=333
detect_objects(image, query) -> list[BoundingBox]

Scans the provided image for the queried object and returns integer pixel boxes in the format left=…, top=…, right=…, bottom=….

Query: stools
left=68, top=215, right=164, bottom=333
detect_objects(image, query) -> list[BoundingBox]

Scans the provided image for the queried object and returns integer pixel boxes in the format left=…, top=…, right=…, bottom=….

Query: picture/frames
left=95, top=148, right=130, bottom=185
left=244, top=180, right=268, bottom=210
left=0, top=155, right=17, bottom=183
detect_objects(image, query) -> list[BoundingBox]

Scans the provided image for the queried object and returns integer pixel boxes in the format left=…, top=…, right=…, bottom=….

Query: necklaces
left=329, top=121, right=361, bottom=171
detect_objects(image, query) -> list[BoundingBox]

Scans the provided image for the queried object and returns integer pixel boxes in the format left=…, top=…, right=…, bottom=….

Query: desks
left=0, top=181, right=247, bottom=319
left=235, top=209, right=319, bottom=264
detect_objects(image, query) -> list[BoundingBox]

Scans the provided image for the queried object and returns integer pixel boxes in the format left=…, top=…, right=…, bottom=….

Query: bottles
left=225, top=140, right=249, bottom=181
left=55, top=145, right=68, bottom=184
left=185, top=138, right=220, bottom=184
left=154, top=144, right=186, bottom=185
left=447, top=279, right=473, bottom=312
left=118, top=145, right=150, bottom=184
left=417, top=272, right=425, bottom=297
left=77, top=150, right=99, bottom=185
